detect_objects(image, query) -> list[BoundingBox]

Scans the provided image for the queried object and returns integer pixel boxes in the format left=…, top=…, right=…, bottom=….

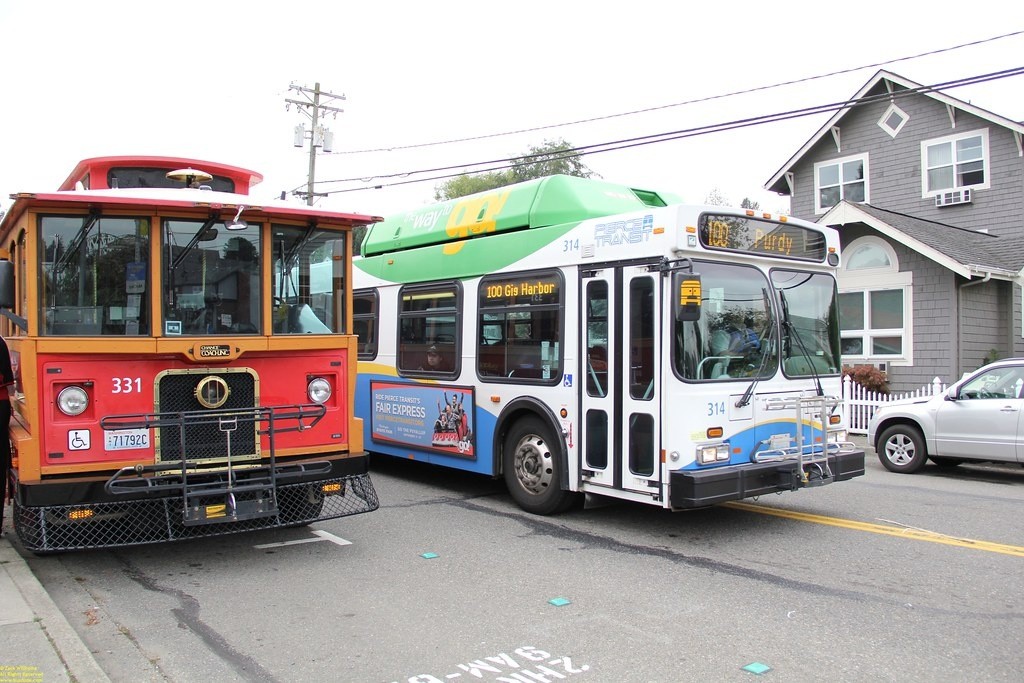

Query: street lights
left=285, top=82, right=345, bottom=206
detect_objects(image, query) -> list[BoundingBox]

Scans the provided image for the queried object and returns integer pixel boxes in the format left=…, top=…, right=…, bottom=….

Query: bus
left=2, top=156, right=386, bottom=557
left=278, top=173, right=867, bottom=516
left=868, top=357, right=1024, bottom=473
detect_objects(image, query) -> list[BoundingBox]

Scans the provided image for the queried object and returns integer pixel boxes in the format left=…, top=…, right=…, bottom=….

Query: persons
left=416, top=344, right=450, bottom=372
left=490, top=322, right=519, bottom=346
left=0, top=329, right=19, bottom=537
left=708, top=297, right=762, bottom=368
